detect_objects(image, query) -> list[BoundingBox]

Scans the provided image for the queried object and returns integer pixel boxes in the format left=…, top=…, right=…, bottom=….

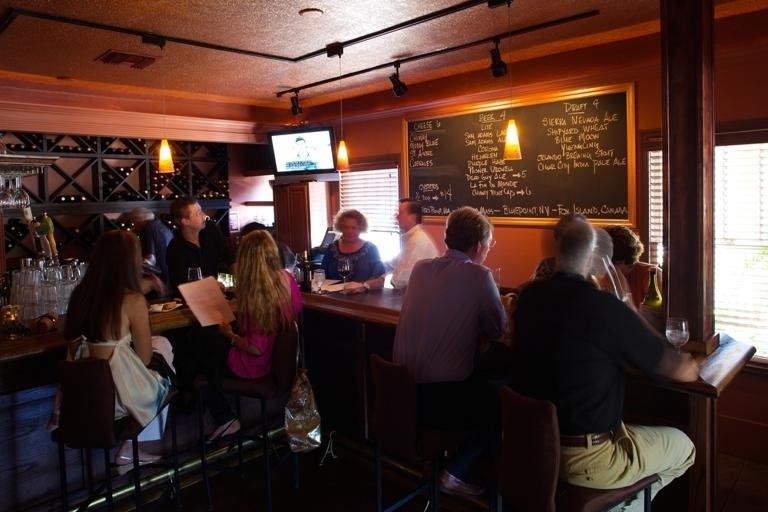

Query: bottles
left=8, top=134, right=43, bottom=151
left=48, top=136, right=230, bottom=248
left=7, top=225, right=24, bottom=249
left=217, top=274, right=233, bottom=288
left=643, top=267, right=662, bottom=314
left=300, top=250, right=311, bottom=292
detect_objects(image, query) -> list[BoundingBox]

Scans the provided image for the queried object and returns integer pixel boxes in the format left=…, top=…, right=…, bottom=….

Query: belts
left=566, top=434, right=611, bottom=446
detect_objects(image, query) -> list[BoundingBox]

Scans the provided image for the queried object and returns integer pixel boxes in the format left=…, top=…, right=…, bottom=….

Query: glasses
left=484, top=238, right=496, bottom=248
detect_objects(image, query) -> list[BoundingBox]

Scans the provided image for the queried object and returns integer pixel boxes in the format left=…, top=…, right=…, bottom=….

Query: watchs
left=362, top=281, right=370, bottom=292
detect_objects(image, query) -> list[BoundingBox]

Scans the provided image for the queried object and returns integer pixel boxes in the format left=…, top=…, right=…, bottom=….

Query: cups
left=313, top=269, right=325, bottom=289
left=9, top=257, right=87, bottom=321
left=490, top=267, right=501, bottom=294
left=188, top=266, right=202, bottom=281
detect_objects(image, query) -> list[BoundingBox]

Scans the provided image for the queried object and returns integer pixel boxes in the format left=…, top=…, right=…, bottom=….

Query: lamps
left=153, top=77, right=178, bottom=174
left=497, top=1, right=527, bottom=165
left=324, top=43, right=354, bottom=176
left=269, top=31, right=510, bottom=117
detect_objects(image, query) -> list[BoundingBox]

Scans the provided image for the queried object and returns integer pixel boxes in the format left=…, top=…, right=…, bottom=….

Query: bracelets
left=231, top=335, right=239, bottom=346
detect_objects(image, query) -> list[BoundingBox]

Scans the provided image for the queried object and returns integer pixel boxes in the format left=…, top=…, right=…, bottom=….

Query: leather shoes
left=440, top=472, right=487, bottom=498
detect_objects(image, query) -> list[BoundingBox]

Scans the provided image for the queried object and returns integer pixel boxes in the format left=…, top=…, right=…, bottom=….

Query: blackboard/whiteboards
left=400, top=81, right=635, bottom=231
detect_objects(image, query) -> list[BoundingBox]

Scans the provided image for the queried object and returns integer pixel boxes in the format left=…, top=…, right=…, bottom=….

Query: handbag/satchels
left=286, top=371, right=322, bottom=453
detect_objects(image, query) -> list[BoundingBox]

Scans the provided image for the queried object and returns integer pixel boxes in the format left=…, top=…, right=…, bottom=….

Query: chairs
left=363, top=353, right=451, bottom=512
left=199, top=318, right=305, bottom=503
left=51, top=356, right=180, bottom=512
left=498, top=387, right=663, bottom=512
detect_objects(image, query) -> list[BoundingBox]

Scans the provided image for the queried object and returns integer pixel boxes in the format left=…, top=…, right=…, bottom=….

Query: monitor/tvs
left=267, top=127, right=336, bottom=176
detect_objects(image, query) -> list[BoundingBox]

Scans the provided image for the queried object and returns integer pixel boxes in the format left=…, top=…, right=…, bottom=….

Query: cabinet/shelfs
left=0, top=205, right=230, bottom=272
left=0, top=130, right=232, bottom=213
left=272, top=182, right=329, bottom=256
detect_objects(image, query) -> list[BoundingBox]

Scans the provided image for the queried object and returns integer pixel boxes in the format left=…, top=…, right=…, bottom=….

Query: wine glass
left=0, top=168, right=32, bottom=209
left=665, top=317, right=690, bottom=357
left=337, top=258, right=352, bottom=294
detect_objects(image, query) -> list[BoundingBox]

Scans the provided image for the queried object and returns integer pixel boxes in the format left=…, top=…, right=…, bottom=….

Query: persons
left=508, top=211, right=700, bottom=512
left=382, top=198, right=440, bottom=288
left=599, top=225, right=663, bottom=324
left=49, top=229, right=178, bottom=477
left=393, top=204, right=509, bottom=511
left=230, top=221, right=300, bottom=281
left=164, top=195, right=237, bottom=297
left=319, top=208, right=386, bottom=295
left=207, top=227, right=305, bottom=447
left=129, top=206, right=174, bottom=274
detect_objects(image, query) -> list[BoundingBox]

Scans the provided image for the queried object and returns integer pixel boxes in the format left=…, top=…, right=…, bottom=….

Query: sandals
left=202, top=420, right=240, bottom=454
left=116, top=453, right=162, bottom=475
